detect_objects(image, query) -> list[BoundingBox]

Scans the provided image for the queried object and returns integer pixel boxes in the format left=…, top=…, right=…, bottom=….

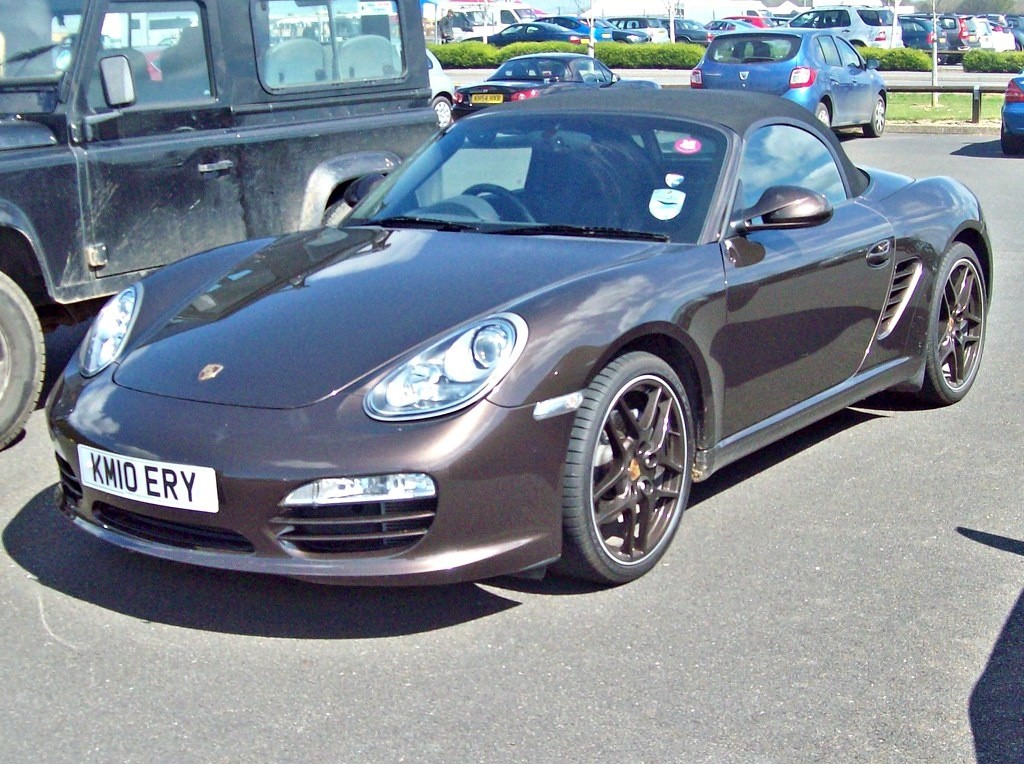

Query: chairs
left=752, top=42, right=771, bottom=58
left=262, top=34, right=402, bottom=88
left=511, top=63, right=526, bottom=77
left=550, top=64, right=565, bottom=77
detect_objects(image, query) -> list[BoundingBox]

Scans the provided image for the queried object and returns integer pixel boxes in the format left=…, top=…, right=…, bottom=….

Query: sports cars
left=42, top=89, right=994, bottom=595
left=449, top=53, right=663, bottom=132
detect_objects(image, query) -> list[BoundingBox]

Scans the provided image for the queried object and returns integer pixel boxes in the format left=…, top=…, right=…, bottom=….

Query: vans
left=446, top=4, right=538, bottom=46
left=0, top=0, right=448, bottom=452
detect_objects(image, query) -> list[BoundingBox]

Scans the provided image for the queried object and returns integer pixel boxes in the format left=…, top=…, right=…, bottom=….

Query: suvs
left=782, top=5, right=906, bottom=52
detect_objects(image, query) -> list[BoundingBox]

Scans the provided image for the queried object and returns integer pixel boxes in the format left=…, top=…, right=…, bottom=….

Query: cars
left=417, top=45, right=458, bottom=133
left=688, top=27, right=889, bottom=139
left=458, top=22, right=591, bottom=51
left=529, top=16, right=613, bottom=46
left=600, top=15, right=823, bottom=45
left=143, top=48, right=167, bottom=82
left=894, top=13, right=1024, bottom=64
left=577, top=17, right=652, bottom=50
left=1000, top=68, right=1024, bottom=156
